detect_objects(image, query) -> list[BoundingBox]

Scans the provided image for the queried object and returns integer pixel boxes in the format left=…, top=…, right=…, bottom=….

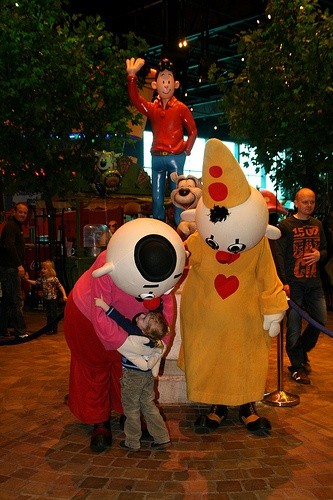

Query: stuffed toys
left=179, top=138, right=288, bottom=435
left=63, top=218, right=190, bottom=453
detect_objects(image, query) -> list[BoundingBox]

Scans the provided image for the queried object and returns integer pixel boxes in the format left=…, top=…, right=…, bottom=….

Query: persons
left=24, top=260, right=68, bottom=334
left=100, top=221, right=118, bottom=245
left=0, top=202, right=31, bottom=340
left=269, top=188, right=330, bottom=386
left=94, top=293, right=171, bottom=453
left=126, top=57, right=198, bottom=227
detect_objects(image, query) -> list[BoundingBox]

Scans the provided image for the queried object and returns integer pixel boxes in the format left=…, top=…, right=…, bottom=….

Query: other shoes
left=150, top=441, right=172, bottom=450
left=47, top=329, right=56, bottom=335
left=16, top=332, right=33, bottom=340
left=302, top=362, right=313, bottom=374
left=290, top=368, right=311, bottom=385
left=120, top=440, right=140, bottom=452
